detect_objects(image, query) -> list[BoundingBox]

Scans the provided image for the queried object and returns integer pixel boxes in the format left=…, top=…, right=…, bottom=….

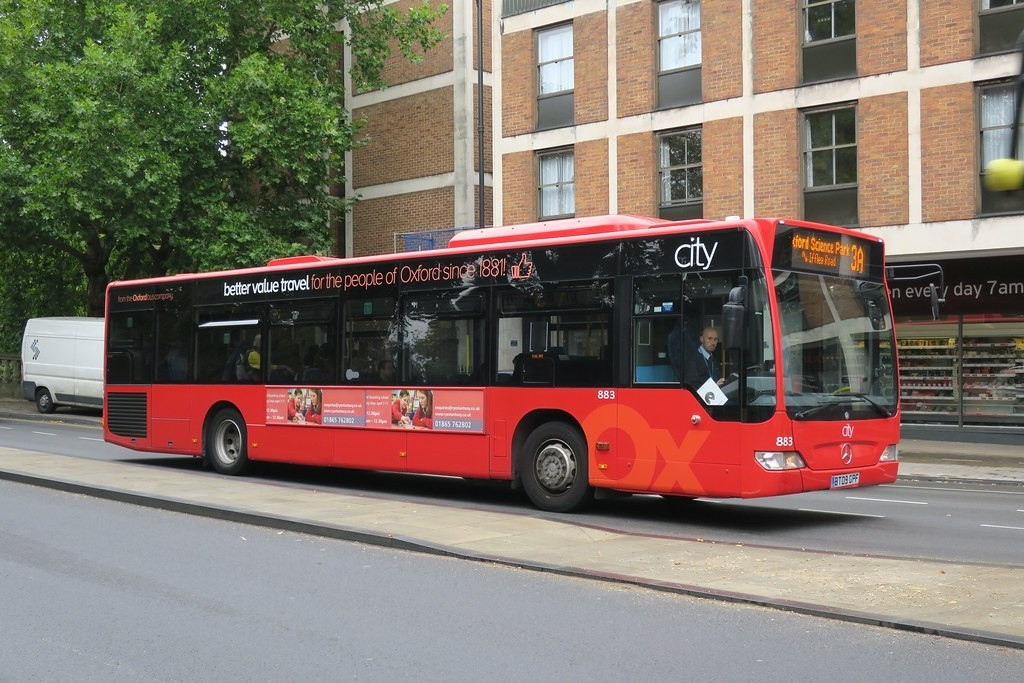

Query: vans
left=20, top=317, right=105, bottom=413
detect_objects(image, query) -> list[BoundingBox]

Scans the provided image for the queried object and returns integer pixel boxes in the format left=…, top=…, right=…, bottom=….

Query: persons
left=392, top=389, right=433, bottom=429
left=288, top=388, right=322, bottom=424
left=235, top=333, right=397, bottom=385
left=683, top=328, right=725, bottom=387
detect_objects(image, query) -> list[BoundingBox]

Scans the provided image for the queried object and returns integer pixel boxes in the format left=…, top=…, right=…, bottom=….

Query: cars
left=822, top=363, right=870, bottom=395
left=986, top=366, right=1024, bottom=400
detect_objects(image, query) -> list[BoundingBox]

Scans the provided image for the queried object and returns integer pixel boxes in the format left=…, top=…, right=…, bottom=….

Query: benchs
left=110, top=312, right=143, bottom=348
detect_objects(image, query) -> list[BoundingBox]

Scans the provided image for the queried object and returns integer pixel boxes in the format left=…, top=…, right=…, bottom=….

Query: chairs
left=230, top=360, right=445, bottom=385
left=666, top=327, right=697, bottom=383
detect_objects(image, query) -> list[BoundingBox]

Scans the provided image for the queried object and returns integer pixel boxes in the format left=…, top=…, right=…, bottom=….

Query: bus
left=101, top=215, right=946, bottom=513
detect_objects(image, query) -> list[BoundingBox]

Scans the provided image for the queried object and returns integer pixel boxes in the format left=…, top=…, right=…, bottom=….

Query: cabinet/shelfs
left=837, top=322, right=1024, bottom=423
